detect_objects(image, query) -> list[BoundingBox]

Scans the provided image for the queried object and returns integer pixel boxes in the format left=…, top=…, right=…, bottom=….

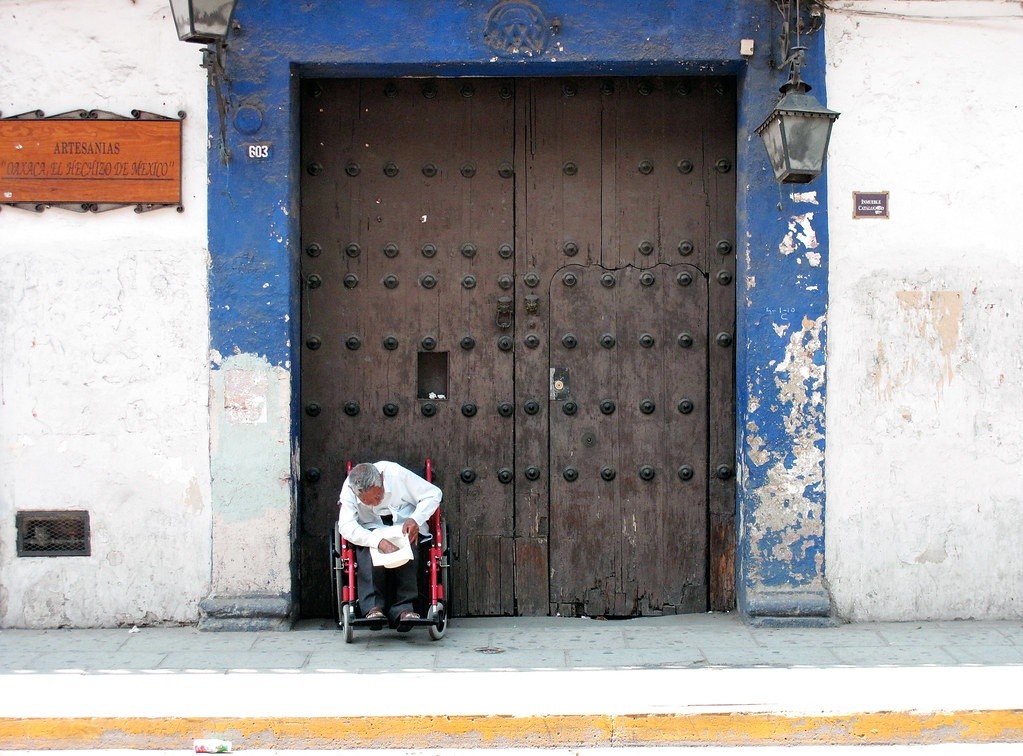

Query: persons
left=337, top=460, right=444, bottom=631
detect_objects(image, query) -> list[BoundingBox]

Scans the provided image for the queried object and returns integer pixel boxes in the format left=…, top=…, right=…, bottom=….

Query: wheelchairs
left=329, top=458, right=452, bottom=643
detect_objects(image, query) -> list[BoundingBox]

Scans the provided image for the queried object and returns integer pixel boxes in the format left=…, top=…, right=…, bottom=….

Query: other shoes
left=366, top=607, right=385, bottom=631
left=397, top=609, right=420, bottom=632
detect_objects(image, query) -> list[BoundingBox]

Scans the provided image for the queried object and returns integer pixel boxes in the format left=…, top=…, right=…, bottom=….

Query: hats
left=369, top=525, right=414, bottom=568
